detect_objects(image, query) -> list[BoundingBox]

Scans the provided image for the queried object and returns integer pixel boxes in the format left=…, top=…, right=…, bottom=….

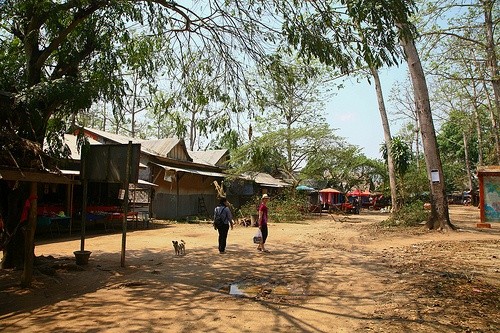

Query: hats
left=261, top=194, right=270, bottom=199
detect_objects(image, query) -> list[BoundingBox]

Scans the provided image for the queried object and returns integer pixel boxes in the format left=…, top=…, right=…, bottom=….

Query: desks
left=37, top=213, right=72, bottom=239
left=84, top=209, right=138, bottom=232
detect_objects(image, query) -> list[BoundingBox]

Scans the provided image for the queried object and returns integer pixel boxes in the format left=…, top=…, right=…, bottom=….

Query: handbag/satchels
left=252, top=228, right=263, bottom=244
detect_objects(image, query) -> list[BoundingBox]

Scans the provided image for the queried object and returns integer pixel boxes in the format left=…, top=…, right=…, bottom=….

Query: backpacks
left=214, top=205, right=227, bottom=229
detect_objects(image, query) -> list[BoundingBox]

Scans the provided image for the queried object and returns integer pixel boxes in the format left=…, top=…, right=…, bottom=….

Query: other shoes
left=220, top=252, right=227, bottom=254
left=263, top=250, right=272, bottom=254
left=257, top=248, right=262, bottom=251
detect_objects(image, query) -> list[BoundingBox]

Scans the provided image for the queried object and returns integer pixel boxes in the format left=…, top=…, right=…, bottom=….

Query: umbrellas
left=319, top=187, right=341, bottom=205
left=297, top=186, right=315, bottom=199
left=346, top=189, right=371, bottom=208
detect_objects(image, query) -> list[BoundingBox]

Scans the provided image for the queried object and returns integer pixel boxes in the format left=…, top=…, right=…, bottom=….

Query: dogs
left=172, top=240, right=186, bottom=255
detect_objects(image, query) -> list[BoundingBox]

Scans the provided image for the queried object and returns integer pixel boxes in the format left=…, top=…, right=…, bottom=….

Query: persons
left=257, top=194, right=270, bottom=252
left=213, top=198, right=233, bottom=254
